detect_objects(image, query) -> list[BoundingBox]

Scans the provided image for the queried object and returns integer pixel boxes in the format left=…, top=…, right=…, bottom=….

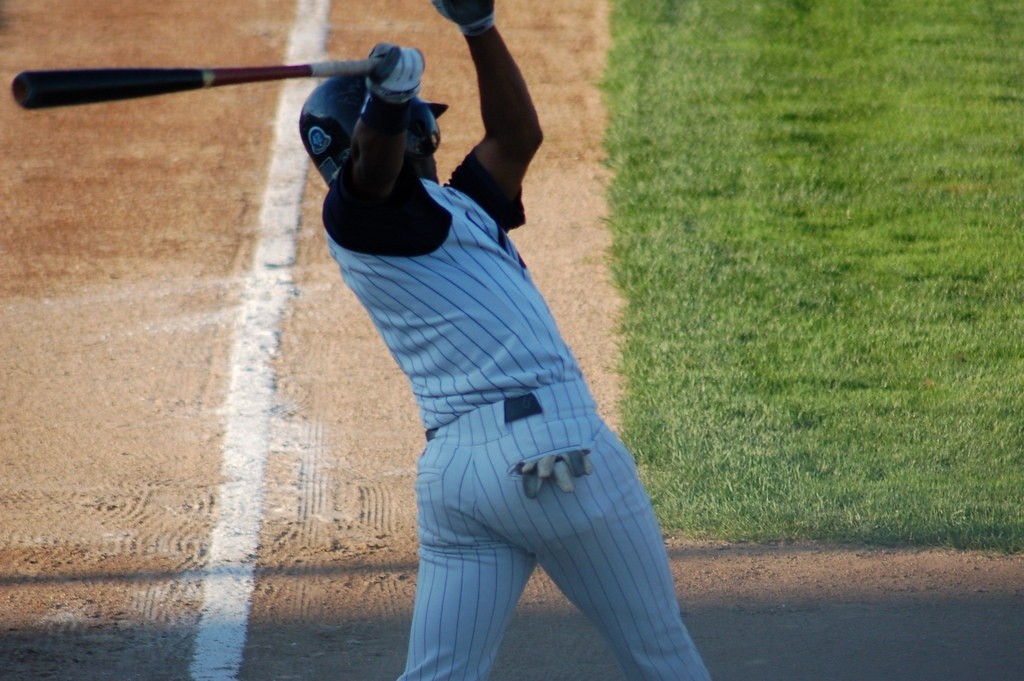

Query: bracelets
left=460, top=12, right=495, bottom=37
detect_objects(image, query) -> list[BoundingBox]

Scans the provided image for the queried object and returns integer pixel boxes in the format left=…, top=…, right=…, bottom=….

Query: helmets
left=299, top=74, right=448, bottom=186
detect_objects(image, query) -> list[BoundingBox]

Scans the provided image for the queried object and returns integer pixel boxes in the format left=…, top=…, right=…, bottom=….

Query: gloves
left=431, top=0, right=497, bottom=35
left=363, top=42, right=425, bottom=102
left=512, top=450, right=587, bottom=498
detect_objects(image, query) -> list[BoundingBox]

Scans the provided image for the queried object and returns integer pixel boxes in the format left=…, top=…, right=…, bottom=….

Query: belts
left=427, top=393, right=542, bottom=440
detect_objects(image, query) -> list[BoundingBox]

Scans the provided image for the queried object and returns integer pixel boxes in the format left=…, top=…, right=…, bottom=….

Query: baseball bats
left=10, top=58, right=385, bottom=110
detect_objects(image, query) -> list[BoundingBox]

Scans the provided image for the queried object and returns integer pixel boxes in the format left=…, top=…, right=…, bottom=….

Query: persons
left=299, top=0, right=711, bottom=680
